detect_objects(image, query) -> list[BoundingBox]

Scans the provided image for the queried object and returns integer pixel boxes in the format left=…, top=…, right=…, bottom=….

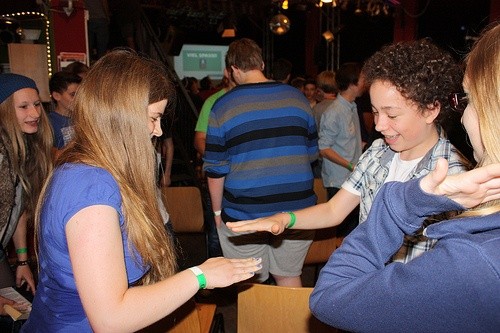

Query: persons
left=268, top=56, right=338, bottom=121
left=20, top=48, right=263, bottom=333
left=0, top=73, right=57, bottom=214
left=309, top=25, right=500, bottom=333
left=0, top=119, right=36, bottom=316
left=46, top=61, right=89, bottom=164
left=159, top=65, right=237, bottom=187
left=225, top=40, right=475, bottom=265
left=317, top=60, right=367, bottom=241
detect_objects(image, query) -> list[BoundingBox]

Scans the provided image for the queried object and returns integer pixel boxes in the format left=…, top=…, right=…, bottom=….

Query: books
left=0, top=286, right=32, bottom=321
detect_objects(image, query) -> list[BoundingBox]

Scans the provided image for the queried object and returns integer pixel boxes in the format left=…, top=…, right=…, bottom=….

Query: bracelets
left=282, top=211, right=296, bottom=228
left=14, top=247, right=28, bottom=255
left=16, top=260, right=31, bottom=265
left=189, top=266, right=207, bottom=289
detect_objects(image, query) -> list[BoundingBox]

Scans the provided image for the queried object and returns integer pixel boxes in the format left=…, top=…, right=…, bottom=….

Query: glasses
left=448, top=92, right=474, bottom=114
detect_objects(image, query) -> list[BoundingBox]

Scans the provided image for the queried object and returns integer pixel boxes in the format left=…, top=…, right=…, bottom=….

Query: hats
left=0, top=73, right=38, bottom=103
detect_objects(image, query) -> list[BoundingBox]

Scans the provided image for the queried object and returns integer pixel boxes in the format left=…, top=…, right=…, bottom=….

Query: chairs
left=150, top=295, right=224, bottom=333
left=238, top=283, right=337, bottom=333
left=302, top=179, right=336, bottom=282
left=160, top=187, right=209, bottom=259
